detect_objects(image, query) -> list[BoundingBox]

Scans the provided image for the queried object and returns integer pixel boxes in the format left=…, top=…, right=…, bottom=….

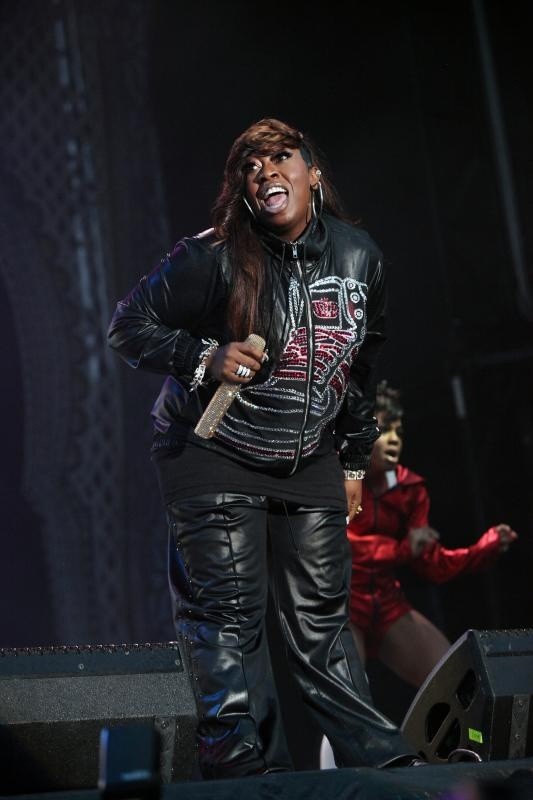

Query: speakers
left=398, top=628, right=533, bottom=767
left=0, top=643, right=196, bottom=793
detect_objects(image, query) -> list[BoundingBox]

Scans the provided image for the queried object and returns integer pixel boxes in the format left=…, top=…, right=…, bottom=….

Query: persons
left=319, top=381, right=517, bottom=771
left=103, top=117, right=424, bottom=780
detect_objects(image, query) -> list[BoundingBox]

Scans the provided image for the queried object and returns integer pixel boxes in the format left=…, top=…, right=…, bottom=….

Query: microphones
left=195, top=334, right=266, bottom=439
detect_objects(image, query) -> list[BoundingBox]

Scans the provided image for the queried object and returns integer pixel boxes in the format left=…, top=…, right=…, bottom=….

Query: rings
left=352, top=504, right=362, bottom=515
left=236, top=365, right=251, bottom=378
